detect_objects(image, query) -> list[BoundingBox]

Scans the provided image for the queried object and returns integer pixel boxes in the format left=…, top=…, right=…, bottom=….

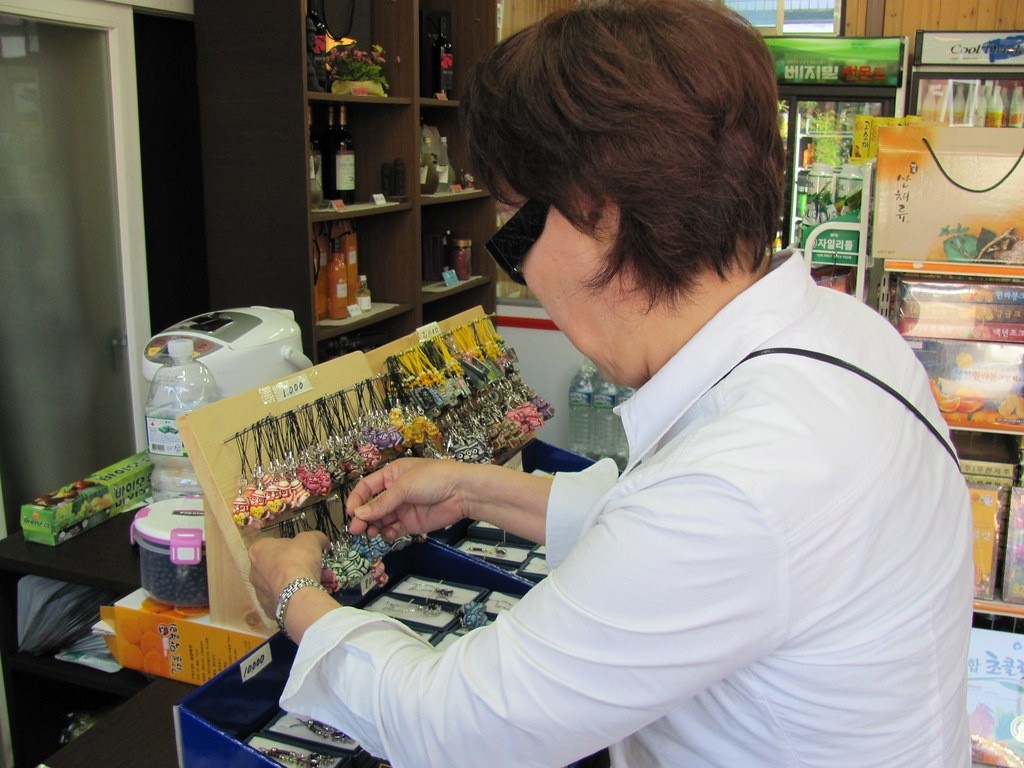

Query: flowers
left=325, top=42, right=403, bottom=94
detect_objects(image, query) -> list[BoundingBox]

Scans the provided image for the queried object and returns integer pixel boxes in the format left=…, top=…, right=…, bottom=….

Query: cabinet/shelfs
left=0, top=503, right=147, bottom=768
left=872, top=257, right=1024, bottom=631
left=193, top=0, right=498, bottom=368
left=804, top=162, right=878, bottom=301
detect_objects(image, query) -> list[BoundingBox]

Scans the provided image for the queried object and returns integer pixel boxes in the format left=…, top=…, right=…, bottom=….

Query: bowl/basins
left=131, top=497, right=209, bottom=607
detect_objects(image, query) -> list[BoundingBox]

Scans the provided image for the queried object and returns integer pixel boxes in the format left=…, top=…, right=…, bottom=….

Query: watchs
left=274, top=577, right=328, bottom=643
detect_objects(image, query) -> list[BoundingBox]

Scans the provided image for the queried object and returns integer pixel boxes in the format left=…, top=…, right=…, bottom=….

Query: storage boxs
left=91, top=585, right=272, bottom=686
left=173, top=435, right=629, bottom=768
left=965, top=627, right=1024, bottom=768
left=21, top=450, right=152, bottom=545
left=894, top=276, right=1024, bottom=433
left=956, top=457, right=1023, bottom=605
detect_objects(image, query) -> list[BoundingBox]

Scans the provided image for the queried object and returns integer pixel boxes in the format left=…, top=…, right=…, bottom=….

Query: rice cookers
left=144, top=306, right=313, bottom=399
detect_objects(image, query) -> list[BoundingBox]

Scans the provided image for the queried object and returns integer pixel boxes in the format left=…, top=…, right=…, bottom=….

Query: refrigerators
left=762, top=35, right=910, bottom=320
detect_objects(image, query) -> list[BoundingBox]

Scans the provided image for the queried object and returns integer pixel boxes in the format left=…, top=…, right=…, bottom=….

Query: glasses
left=484, top=191, right=552, bottom=285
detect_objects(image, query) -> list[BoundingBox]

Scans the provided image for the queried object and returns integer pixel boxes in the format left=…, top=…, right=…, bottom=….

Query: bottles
left=568, top=362, right=640, bottom=465
left=355, top=275, right=371, bottom=311
left=918, top=79, right=1023, bottom=129
left=452, top=238, right=473, bottom=280
left=759, top=101, right=882, bottom=266
left=143, top=337, right=221, bottom=502
left=327, top=238, right=347, bottom=321
left=309, top=104, right=358, bottom=206
left=433, top=16, right=456, bottom=100
left=421, top=136, right=454, bottom=195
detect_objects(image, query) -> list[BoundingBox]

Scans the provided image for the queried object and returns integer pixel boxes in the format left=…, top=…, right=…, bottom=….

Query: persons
left=247, top=0, right=973, bottom=768
